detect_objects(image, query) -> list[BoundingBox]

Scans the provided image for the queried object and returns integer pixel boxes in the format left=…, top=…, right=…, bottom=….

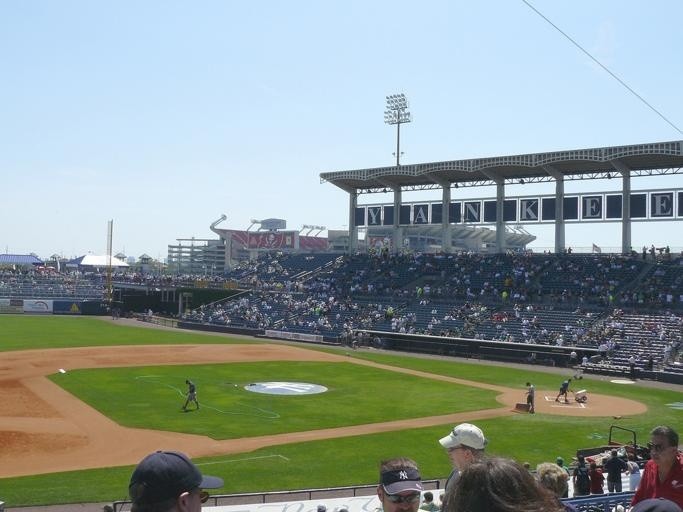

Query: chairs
left=0, top=249, right=683, bottom=386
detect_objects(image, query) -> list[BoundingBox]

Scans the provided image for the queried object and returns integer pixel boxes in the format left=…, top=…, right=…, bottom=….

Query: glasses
left=184, top=490, right=210, bottom=504
left=379, top=487, right=421, bottom=503
left=646, top=440, right=676, bottom=451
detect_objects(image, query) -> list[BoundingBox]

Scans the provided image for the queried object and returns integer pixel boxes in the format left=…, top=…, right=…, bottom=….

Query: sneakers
left=554, top=398, right=570, bottom=404
left=182, top=405, right=201, bottom=410
left=529, top=408, right=535, bottom=413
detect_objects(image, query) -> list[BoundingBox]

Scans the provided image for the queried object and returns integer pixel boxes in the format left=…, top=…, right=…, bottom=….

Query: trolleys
left=568, top=388, right=589, bottom=403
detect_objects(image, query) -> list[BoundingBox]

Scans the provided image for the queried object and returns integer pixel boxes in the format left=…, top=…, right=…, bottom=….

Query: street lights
left=384, top=93, right=412, bottom=167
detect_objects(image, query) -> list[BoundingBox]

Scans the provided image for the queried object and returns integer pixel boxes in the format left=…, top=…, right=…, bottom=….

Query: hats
left=567, top=378, right=572, bottom=382
left=525, top=382, right=530, bottom=387
left=437, top=422, right=486, bottom=449
left=128, top=448, right=225, bottom=502
left=316, top=502, right=348, bottom=512
left=553, top=451, right=635, bottom=465
left=185, top=378, right=191, bottom=384
left=378, top=467, right=425, bottom=495
left=629, top=497, right=683, bottom=512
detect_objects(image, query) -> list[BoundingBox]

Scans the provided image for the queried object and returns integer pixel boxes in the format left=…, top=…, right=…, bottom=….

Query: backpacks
left=576, top=468, right=590, bottom=491
